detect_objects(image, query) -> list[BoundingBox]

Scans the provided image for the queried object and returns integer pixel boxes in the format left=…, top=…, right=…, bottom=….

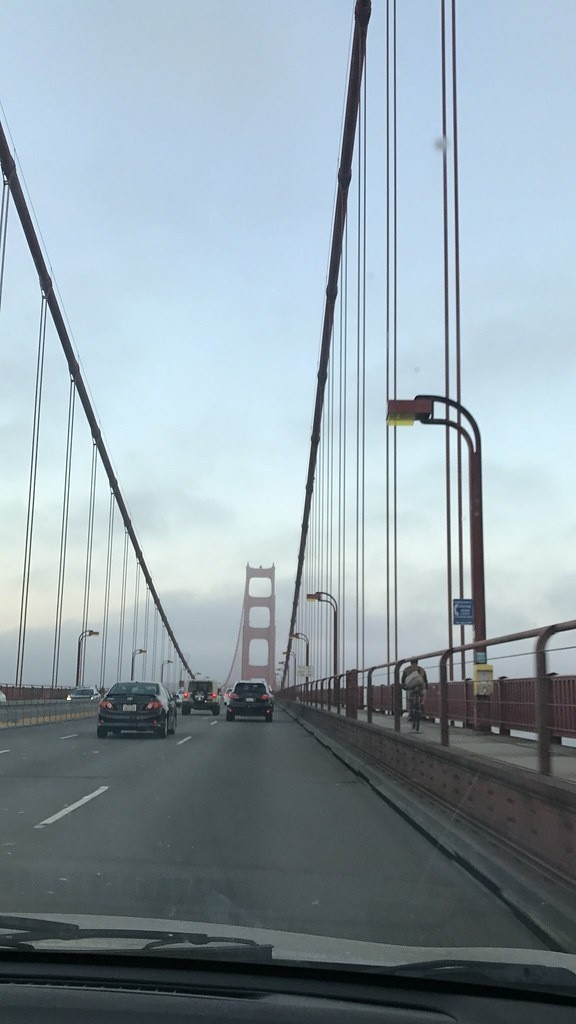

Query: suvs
left=182, top=678, right=221, bottom=716
left=225, top=681, right=273, bottom=722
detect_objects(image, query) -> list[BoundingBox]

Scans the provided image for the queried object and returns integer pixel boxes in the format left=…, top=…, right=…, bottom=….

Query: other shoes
left=408, top=717, right=412, bottom=722
left=421, top=703, right=425, bottom=713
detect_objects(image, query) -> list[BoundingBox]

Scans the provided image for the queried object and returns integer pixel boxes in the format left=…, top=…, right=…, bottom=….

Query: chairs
left=256, top=686, right=265, bottom=693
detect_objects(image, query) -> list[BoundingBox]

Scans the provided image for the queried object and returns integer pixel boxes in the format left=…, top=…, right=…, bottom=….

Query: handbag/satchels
left=404, top=666, right=423, bottom=689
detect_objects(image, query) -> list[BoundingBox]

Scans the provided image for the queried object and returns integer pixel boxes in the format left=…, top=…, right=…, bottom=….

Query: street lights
left=131, top=649, right=147, bottom=681
left=307, top=590, right=339, bottom=704
left=161, top=660, right=173, bottom=683
left=280, top=660, right=290, bottom=688
left=385, top=393, right=492, bottom=730
left=289, top=632, right=310, bottom=692
left=283, top=651, right=297, bottom=686
left=76, top=629, right=99, bottom=687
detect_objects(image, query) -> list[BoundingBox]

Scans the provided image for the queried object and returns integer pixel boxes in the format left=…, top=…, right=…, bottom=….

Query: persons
left=400, top=656, right=429, bottom=721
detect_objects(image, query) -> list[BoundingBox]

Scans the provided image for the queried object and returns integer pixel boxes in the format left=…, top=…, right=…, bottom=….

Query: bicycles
left=404, top=686, right=427, bottom=731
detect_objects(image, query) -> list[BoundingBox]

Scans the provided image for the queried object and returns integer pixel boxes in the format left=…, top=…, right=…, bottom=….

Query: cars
left=176, top=689, right=184, bottom=704
left=97, top=681, right=179, bottom=738
left=224, top=688, right=233, bottom=704
left=0, top=690, right=6, bottom=703
left=67, top=687, right=101, bottom=701
